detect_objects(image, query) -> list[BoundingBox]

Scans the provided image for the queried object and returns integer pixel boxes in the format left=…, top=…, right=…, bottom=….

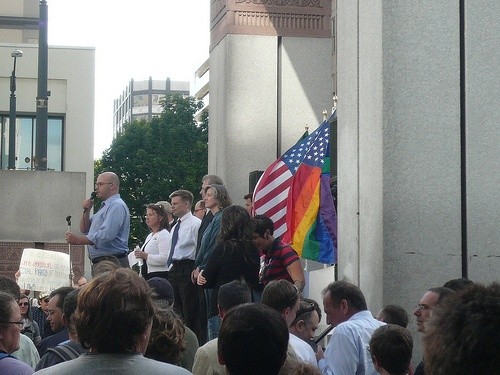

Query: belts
left=92, top=253, right=127, bottom=264
left=174, top=259, right=190, bottom=265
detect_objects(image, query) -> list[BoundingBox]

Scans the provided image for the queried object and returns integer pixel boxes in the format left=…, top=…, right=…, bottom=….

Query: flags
left=252, top=121, right=326, bottom=264
left=280, top=110, right=337, bottom=264
left=293, top=132, right=306, bottom=145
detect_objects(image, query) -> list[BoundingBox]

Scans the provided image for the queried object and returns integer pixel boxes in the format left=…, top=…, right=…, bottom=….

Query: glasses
left=19, top=302, right=28, bottom=306
left=246, top=204, right=249, bottom=206
left=95, top=182, right=111, bottom=186
left=44, top=300, right=49, bottom=303
left=290, top=284, right=299, bottom=301
left=155, top=202, right=162, bottom=209
left=416, top=305, right=426, bottom=312
left=7, top=320, right=24, bottom=328
left=300, top=303, right=315, bottom=314
left=194, top=208, right=203, bottom=212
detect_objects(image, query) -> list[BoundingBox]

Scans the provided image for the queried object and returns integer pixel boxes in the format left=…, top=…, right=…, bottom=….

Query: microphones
left=83, top=191, right=96, bottom=213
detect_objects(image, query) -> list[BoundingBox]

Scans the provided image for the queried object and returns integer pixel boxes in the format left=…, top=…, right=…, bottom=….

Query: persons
left=0, top=175, right=500, bottom=375
left=65, top=172, right=130, bottom=280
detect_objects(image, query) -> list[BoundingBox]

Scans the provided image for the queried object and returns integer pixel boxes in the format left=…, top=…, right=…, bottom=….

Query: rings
left=66, top=238, right=67, bottom=240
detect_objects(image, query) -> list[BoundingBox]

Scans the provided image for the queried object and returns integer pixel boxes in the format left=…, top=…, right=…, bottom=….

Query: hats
left=37, top=292, right=49, bottom=305
left=146, top=277, right=175, bottom=301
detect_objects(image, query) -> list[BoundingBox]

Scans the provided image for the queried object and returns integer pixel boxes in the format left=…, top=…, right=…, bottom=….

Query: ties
left=166, top=220, right=181, bottom=267
